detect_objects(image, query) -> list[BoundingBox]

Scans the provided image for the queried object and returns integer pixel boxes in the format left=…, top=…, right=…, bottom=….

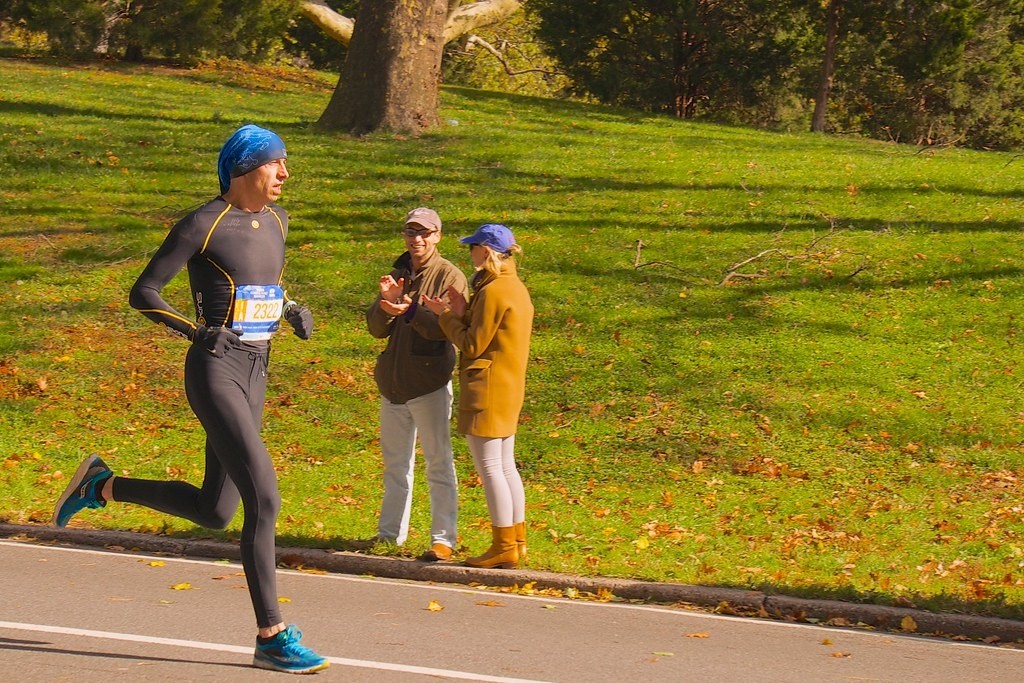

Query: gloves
left=287, top=305, right=314, bottom=340
left=188, top=323, right=244, bottom=359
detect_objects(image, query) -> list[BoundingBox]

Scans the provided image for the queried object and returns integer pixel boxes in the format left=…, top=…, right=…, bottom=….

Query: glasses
left=402, top=228, right=437, bottom=238
left=469, top=243, right=482, bottom=252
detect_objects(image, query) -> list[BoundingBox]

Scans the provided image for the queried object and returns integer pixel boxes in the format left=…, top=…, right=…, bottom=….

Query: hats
left=405, top=207, right=441, bottom=232
left=459, top=224, right=516, bottom=252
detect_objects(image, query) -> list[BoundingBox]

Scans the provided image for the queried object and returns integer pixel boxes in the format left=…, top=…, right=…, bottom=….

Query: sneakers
left=253, top=624, right=330, bottom=673
left=53, top=452, right=113, bottom=529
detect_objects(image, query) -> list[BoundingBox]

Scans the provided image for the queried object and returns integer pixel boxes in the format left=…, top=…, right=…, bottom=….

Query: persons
left=348, top=207, right=467, bottom=561
left=420, top=225, right=534, bottom=568
left=54, top=125, right=333, bottom=675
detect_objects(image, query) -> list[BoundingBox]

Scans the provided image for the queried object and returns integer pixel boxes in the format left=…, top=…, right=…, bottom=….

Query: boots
left=464, top=525, right=518, bottom=568
left=513, top=521, right=530, bottom=566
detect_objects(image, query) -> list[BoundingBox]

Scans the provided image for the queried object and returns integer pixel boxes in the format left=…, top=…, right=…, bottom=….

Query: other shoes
left=349, top=537, right=391, bottom=551
left=424, top=543, right=453, bottom=559
